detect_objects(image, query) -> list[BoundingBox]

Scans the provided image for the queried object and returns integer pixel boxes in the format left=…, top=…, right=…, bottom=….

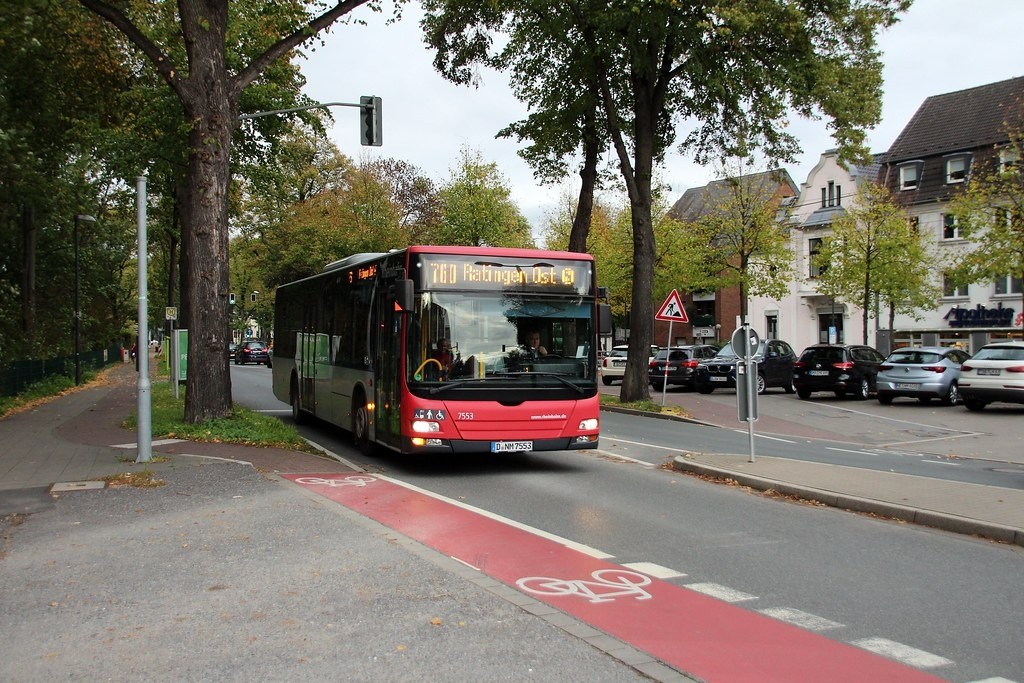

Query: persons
left=434, top=337, right=456, bottom=379
left=519, top=331, right=549, bottom=361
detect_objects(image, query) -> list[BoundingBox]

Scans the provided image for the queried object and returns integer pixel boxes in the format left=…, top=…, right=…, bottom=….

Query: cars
left=229, top=343, right=237, bottom=356
left=876, top=346, right=972, bottom=405
left=957, top=341, right=1024, bottom=410
left=649, top=345, right=720, bottom=391
left=233, top=340, right=268, bottom=365
left=792, top=343, right=886, bottom=400
left=696, top=340, right=797, bottom=393
left=601, top=345, right=660, bottom=386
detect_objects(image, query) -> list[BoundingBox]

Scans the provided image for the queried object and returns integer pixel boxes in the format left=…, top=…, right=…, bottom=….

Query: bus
left=272, top=246, right=615, bottom=458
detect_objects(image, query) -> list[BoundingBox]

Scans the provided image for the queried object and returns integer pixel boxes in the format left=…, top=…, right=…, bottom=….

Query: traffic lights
left=230, top=293, right=236, bottom=304
left=251, top=294, right=256, bottom=302
